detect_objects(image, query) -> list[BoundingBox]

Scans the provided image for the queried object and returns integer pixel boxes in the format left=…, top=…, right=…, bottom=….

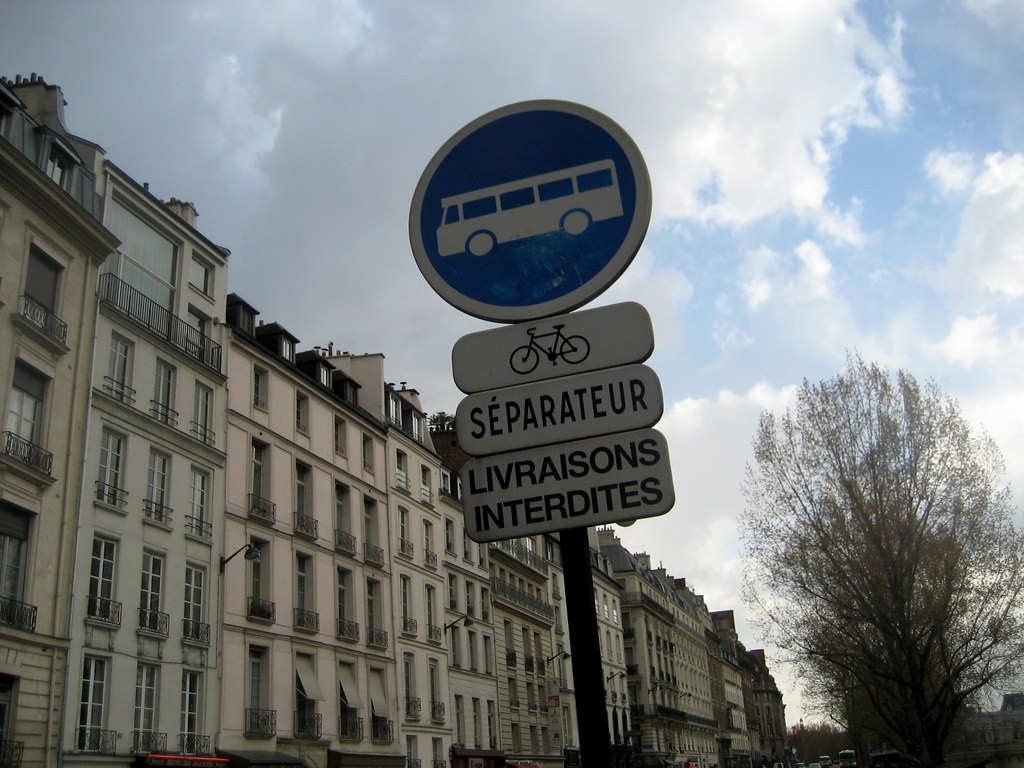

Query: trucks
left=820, top=756, right=830, bottom=768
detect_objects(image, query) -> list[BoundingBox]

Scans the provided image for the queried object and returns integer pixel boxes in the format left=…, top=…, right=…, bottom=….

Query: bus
left=838, top=749, right=855, bottom=768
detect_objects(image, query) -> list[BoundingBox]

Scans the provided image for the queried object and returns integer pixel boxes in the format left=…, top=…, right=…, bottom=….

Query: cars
left=795, top=762, right=806, bottom=768
left=809, top=762, right=821, bottom=768
